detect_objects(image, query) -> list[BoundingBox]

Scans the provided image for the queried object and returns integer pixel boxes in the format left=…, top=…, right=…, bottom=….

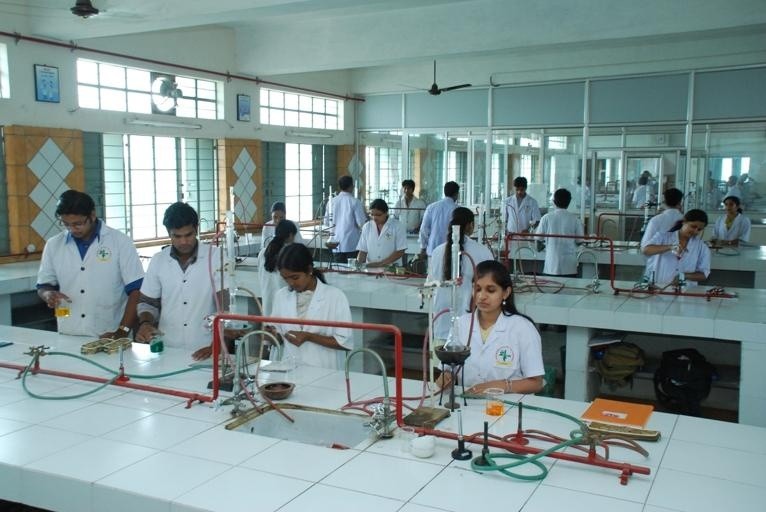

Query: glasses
left=58, top=215, right=89, bottom=228
left=369, top=211, right=384, bottom=217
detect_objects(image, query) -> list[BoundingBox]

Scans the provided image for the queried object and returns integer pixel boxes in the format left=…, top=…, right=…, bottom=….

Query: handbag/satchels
left=654, top=348, right=715, bottom=402
left=599, top=343, right=645, bottom=384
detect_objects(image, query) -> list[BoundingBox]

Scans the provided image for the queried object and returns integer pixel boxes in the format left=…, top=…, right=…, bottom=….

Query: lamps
left=72, top=1, right=101, bottom=19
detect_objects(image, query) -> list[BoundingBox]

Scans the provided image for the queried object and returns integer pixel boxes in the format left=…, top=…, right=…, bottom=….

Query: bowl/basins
left=260, top=382, right=292, bottom=398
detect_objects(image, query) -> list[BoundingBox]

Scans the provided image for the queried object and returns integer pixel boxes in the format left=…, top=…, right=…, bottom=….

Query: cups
left=482, top=387, right=505, bottom=416
left=55, top=295, right=71, bottom=317
left=395, top=265, right=405, bottom=276
left=148, top=331, right=165, bottom=354
left=673, top=246, right=687, bottom=259
left=348, top=258, right=356, bottom=268
left=398, top=429, right=436, bottom=457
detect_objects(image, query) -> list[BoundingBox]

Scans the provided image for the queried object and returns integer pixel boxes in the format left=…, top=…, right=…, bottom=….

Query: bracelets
left=504, top=379, right=513, bottom=393
left=119, top=324, right=130, bottom=333
left=139, top=320, right=151, bottom=325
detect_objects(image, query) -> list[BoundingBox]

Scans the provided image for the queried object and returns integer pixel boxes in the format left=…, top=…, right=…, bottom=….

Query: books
left=579, top=398, right=654, bottom=429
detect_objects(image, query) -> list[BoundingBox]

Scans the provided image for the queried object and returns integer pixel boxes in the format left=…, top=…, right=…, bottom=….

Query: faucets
left=343, top=344, right=394, bottom=438
left=600, top=218, right=616, bottom=241
left=512, top=246, right=536, bottom=279
left=576, top=249, right=599, bottom=293
left=232, top=328, right=281, bottom=413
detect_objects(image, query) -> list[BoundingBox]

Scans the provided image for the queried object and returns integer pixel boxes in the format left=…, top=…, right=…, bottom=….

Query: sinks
left=226, top=402, right=381, bottom=451
left=518, top=285, right=588, bottom=295
left=595, top=245, right=640, bottom=251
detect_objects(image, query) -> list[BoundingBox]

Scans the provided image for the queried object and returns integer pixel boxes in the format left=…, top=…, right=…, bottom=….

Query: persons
left=256, top=243, right=354, bottom=370
left=435, top=260, right=545, bottom=399
left=324, top=176, right=462, bottom=268
left=257, top=221, right=304, bottom=361
left=577, top=176, right=591, bottom=208
left=533, top=189, right=584, bottom=333
left=36, top=190, right=145, bottom=340
left=426, top=207, right=495, bottom=382
left=630, top=169, right=760, bottom=382
left=134, top=202, right=231, bottom=362
left=500, top=177, right=541, bottom=273
left=261, top=201, right=302, bottom=249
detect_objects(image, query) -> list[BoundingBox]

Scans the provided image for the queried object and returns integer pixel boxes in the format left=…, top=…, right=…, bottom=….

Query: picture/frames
left=236, top=93, right=252, bottom=122
left=35, top=63, right=61, bottom=103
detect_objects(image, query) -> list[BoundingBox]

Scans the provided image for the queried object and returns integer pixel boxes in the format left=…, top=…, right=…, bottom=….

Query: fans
left=395, top=59, right=472, bottom=97
